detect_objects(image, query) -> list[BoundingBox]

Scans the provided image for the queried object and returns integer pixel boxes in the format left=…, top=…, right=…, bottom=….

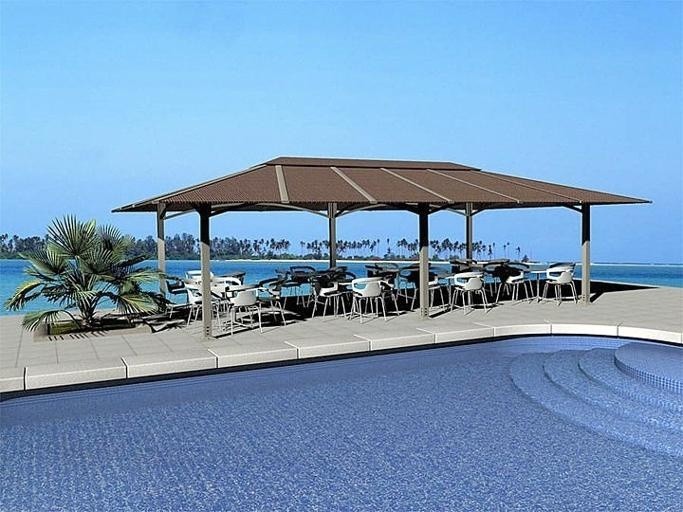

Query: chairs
left=184, top=271, right=286, bottom=336
left=275, top=257, right=577, bottom=324
